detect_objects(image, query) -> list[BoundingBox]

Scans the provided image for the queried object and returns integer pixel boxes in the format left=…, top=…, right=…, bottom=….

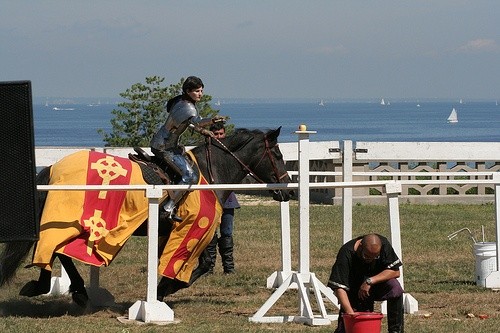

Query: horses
left=0, top=126, right=293, bottom=310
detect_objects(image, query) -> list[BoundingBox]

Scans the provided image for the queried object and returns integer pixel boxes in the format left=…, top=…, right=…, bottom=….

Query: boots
left=218, top=236, right=235, bottom=276
left=202, top=234, right=218, bottom=277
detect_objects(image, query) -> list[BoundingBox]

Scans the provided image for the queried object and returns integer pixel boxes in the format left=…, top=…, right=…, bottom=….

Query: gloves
left=200, top=128, right=214, bottom=138
left=213, top=114, right=226, bottom=124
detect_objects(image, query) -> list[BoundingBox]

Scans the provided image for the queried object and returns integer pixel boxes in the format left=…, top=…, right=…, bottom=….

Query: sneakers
left=160, top=210, right=183, bottom=222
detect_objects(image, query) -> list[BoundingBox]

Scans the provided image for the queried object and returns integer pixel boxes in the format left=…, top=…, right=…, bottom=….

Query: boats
left=52, top=106, right=76, bottom=111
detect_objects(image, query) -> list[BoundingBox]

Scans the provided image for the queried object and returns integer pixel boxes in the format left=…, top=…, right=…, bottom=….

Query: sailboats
left=216, top=100, right=220, bottom=105
left=449, top=108, right=459, bottom=122
left=319, top=100, right=327, bottom=105
left=495, top=101, right=499, bottom=106
left=459, top=99, right=463, bottom=103
left=381, top=99, right=385, bottom=105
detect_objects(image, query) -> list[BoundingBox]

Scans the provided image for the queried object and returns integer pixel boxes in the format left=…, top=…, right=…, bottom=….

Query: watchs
left=366, top=278, right=374, bottom=285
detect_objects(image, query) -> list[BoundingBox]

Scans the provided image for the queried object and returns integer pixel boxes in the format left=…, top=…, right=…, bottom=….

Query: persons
left=327, top=233, right=404, bottom=333
left=150, top=76, right=226, bottom=222
left=202, top=122, right=242, bottom=275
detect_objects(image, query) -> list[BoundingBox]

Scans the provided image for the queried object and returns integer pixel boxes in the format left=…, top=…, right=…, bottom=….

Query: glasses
left=362, top=250, right=380, bottom=260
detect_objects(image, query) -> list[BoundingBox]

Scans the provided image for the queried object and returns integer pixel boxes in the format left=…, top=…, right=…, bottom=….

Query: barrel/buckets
left=474, top=242, right=496, bottom=286
left=342, top=312, right=384, bottom=333
